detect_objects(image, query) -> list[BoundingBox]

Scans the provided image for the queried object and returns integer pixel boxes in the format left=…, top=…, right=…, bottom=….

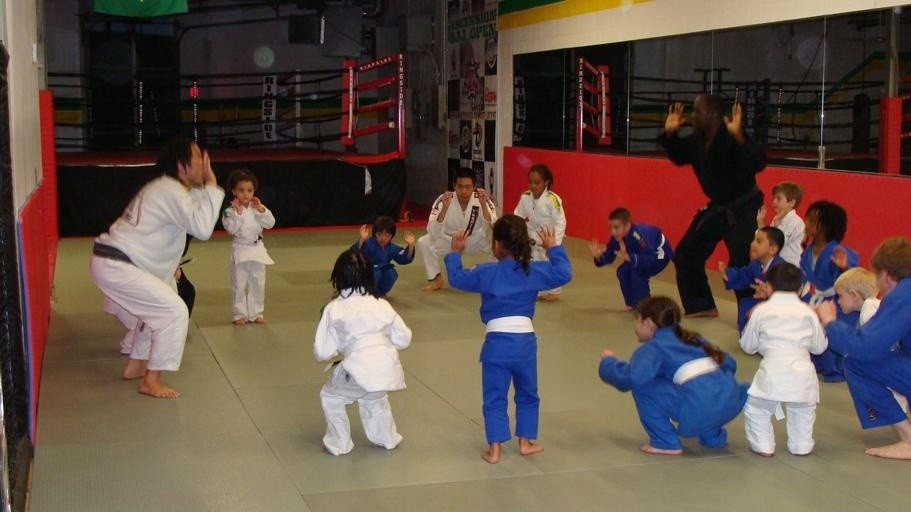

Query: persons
left=217, top=170, right=276, bottom=326
left=721, top=181, right=908, bottom=412
left=351, top=215, right=416, bottom=297
left=508, top=164, right=566, bottom=303
left=416, top=167, right=497, bottom=291
left=590, top=207, right=672, bottom=313
left=444, top=215, right=573, bottom=464
left=739, top=261, right=828, bottom=457
left=309, top=248, right=410, bottom=456
left=598, top=296, right=751, bottom=456
left=91, top=138, right=226, bottom=397
left=817, top=237, right=911, bottom=459
left=658, top=92, right=767, bottom=319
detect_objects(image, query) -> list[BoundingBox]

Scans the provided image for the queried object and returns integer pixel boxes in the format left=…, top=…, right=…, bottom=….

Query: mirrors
left=510, top=3, right=911, bottom=181
